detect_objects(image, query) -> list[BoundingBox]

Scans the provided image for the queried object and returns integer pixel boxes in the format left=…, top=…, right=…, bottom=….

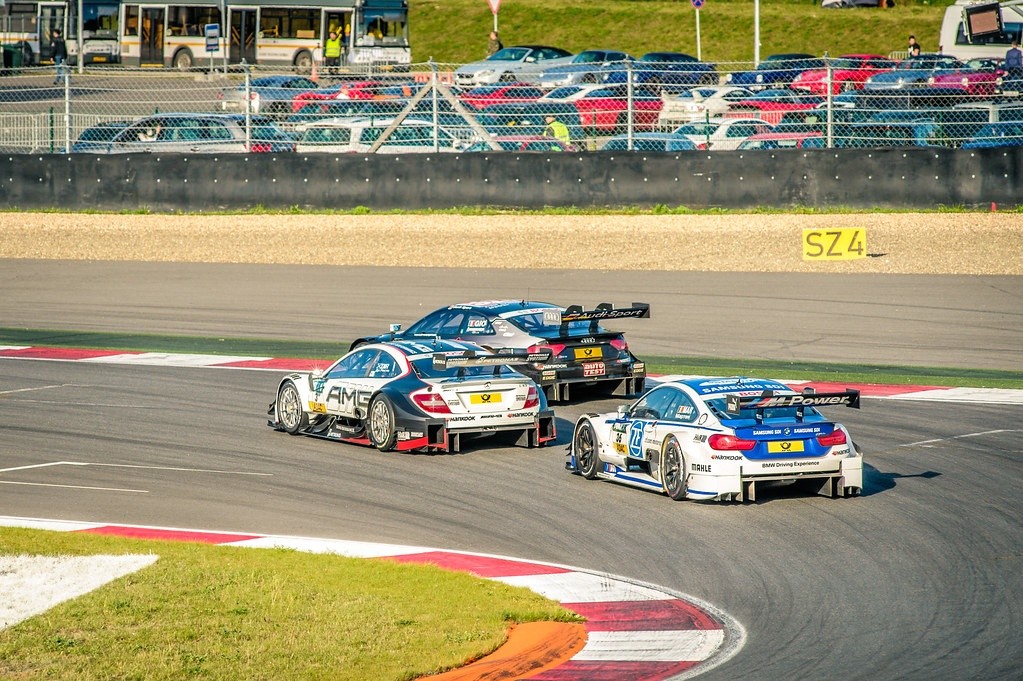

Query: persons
left=545, top=116, right=570, bottom=151
left=486, top=31, right=503, bottom=56
left=323, top=32, right=345, bottom=75
left=139, top=123, right=162, bottom=141
left=337, top=85, right=358, bottom=99
left=1006, top=40, right=1023, bottom=68
left=50, top=29, right=68, bottom=84
left=908, top=35, right=920, bottom=57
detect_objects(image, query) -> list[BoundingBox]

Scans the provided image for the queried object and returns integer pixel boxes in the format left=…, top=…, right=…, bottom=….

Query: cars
left=266, top=322, right=557, bottom=454
left=565, top=374, right=862, bottom=502
left=349, top=300, right=651, bottom=401
left=76, top=42, right=1023, bottom=154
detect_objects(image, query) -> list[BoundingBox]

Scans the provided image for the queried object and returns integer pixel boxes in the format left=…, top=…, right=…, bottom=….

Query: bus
left=118, top=0, right=413, bottom=74
left=0, top=0, right=123, bottom=67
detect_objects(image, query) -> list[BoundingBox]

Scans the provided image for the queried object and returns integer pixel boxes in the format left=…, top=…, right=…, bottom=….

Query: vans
left=938, top=0, right=1023, bottom=63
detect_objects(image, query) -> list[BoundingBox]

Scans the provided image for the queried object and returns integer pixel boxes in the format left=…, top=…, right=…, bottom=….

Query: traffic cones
left=309, top=65, right=320, bottom=80
